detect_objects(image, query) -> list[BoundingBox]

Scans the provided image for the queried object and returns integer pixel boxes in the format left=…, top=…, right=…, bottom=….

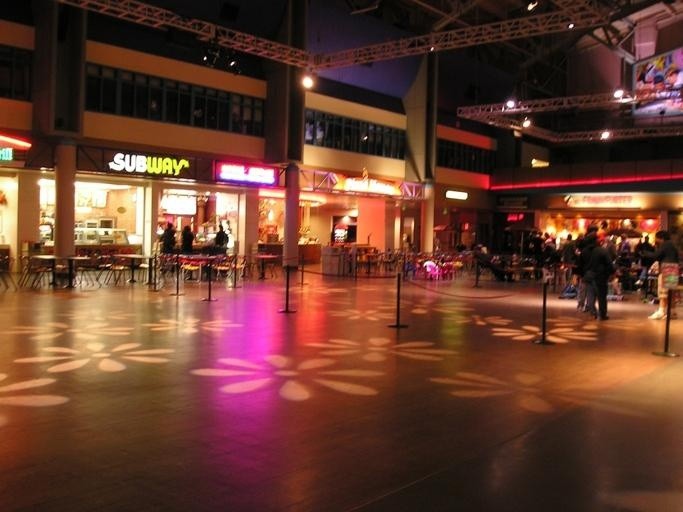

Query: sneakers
left=648, top=312, right=664, bottom=319
left=662, top=313, right=677, bottom=319
left=576, top=304, right=609, bottom=321
left=635, top=280, right=643, bottom=285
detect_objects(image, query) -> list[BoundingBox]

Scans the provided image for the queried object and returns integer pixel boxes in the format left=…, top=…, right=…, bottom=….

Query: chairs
left=0, top=252, right=284, bottom=292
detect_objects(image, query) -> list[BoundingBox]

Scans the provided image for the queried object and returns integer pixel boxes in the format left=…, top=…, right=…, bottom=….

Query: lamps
left=613, top=85, right=625, bottom=99
left=521, top=115, right=530, bottom=127
left=506, top=94, right=517, bottom=109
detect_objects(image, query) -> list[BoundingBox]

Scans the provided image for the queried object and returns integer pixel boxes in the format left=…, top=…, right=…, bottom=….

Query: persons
left=635, top=63, right=683, bottom=110
left=159, top=222, right=177, bottom=273
left=512, top=218, right=680, bottom=321
left=180, top=225, right=195, bottom=280
left=211, top=224, right=229, bottom=278
